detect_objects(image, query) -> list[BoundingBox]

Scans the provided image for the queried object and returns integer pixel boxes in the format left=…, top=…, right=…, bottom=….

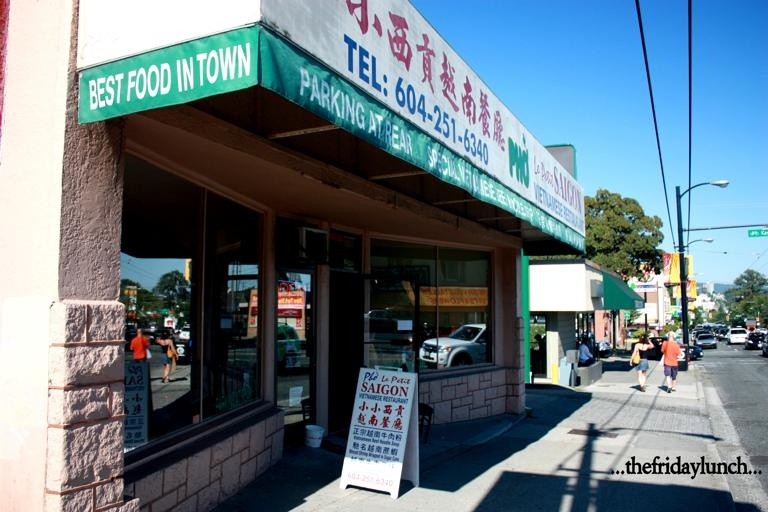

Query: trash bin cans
left=558, top=356, right=577, bottom=387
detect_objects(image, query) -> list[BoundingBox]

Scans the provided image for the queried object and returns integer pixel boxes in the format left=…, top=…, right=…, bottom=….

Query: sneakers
left=667, top=388, right=671, bottom=393
left=673, top=389, right=676, bottom=391
left=641, top=386, right=645, bottom=392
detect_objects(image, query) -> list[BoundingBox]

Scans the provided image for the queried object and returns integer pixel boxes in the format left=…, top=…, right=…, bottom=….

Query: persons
left=628, top=332, right=655, bottom=391
left=660, top=331, right=682, bottom=392
left=130, top=328, right=151, bottom=363
left=577, top=337, right=595, bottom=367
left=156, top=331, right=178, bottom=383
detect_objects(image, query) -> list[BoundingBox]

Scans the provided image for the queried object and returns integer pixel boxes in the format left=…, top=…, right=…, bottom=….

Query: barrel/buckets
left=304, top=424, right=325, bottom=448
left=289, top=386, right=303, bottom=407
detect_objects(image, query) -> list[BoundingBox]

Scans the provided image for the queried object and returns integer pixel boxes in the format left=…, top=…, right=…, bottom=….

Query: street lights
left=673, top=180, right=731, bottom=368
left=685, top=238, right=714, bottom=248
left=700, top=250, right=728, bottom=255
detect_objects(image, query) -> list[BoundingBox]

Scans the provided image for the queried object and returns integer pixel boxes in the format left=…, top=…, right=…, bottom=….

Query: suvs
left=419, top=321, right=486, bottom=368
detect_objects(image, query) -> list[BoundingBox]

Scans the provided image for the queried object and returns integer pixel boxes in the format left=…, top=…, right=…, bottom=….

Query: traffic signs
left=747, top=228, right=768, bottom=238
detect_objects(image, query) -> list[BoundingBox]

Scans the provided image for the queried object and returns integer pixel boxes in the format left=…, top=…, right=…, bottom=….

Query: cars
left=277, top=320, right=310, bottom=373
left=126, top=319, right=191, bottom=366
left=363, top=309, right=408, bottom=334
left=574, top=330, right=594, bottom=341
left=628, top=335, right=703, bottom=361
left=691, top=322, right=767, bottom=358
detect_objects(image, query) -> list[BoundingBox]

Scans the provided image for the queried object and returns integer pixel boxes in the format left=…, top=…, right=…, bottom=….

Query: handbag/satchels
left=167, top=349, right=174, bottom=358
left=633, top=354, right=640, bottom=364
